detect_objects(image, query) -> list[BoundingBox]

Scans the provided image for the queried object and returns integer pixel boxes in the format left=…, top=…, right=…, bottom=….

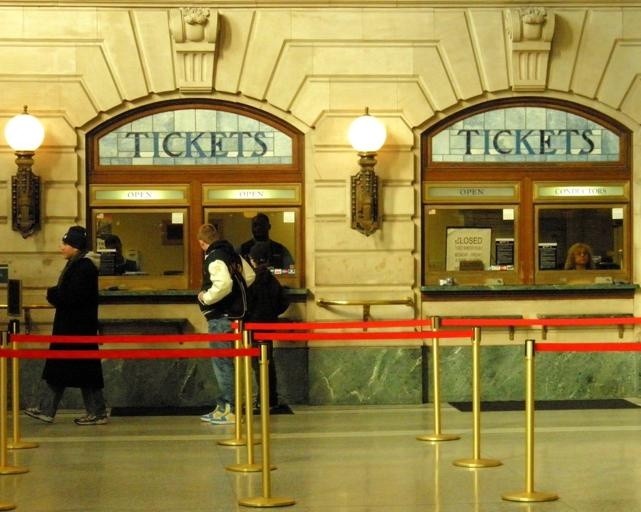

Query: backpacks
left=262, top=278, right=291, bottom=318
left=228, top=251, right=249, bottom=321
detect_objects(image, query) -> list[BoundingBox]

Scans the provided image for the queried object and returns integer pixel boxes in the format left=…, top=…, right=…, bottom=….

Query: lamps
left=4, top=104, right=44, bottom=238
left=349, top=105, right=388, bottom=239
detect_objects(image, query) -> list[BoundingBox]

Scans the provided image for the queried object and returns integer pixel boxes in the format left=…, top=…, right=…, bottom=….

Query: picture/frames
left=161, top=219, right=226, bottom=246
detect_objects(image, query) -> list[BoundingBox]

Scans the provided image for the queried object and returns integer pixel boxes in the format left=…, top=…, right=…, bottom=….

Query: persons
left=236, top=213, right=295, bottom=270
left=241, top=241, right=289, bottom=414
left=564, top=242, right=594, bottom=270
left=196, top=223, right=245, bottom=425
left=104, top=235, right=140, bottom=272
left=24, top=226, right=110, bottom=426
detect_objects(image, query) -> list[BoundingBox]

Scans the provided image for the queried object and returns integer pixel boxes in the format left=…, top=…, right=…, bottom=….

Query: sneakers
left=253, top=395, right=280, bottom=411
left=210, top=403, right=245, bottom=424
left=200, top=401, right=232, bottom=422
left=24, top=408, right=55, bottom=424
left=73, top=410, right=108, bottom=426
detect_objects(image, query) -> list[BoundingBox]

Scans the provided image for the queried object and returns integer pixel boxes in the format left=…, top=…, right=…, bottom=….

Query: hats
left=61, top=226, right=89, bottom=251
left=247, top=243, right=272, bottom=259
